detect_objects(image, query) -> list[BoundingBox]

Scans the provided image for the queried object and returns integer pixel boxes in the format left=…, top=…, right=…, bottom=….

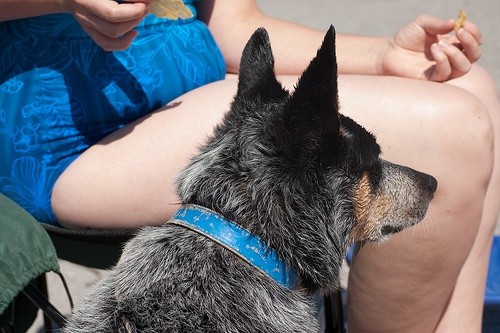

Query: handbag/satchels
left=0, top=192, right=76, bottom=333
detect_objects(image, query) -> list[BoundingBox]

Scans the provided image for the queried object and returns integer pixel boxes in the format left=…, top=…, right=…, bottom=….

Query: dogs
left=53, top=25, right=437, bottom=333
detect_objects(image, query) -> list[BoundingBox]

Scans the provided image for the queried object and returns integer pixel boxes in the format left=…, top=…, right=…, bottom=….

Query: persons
left=0, top=0, right=500, bottom=333
